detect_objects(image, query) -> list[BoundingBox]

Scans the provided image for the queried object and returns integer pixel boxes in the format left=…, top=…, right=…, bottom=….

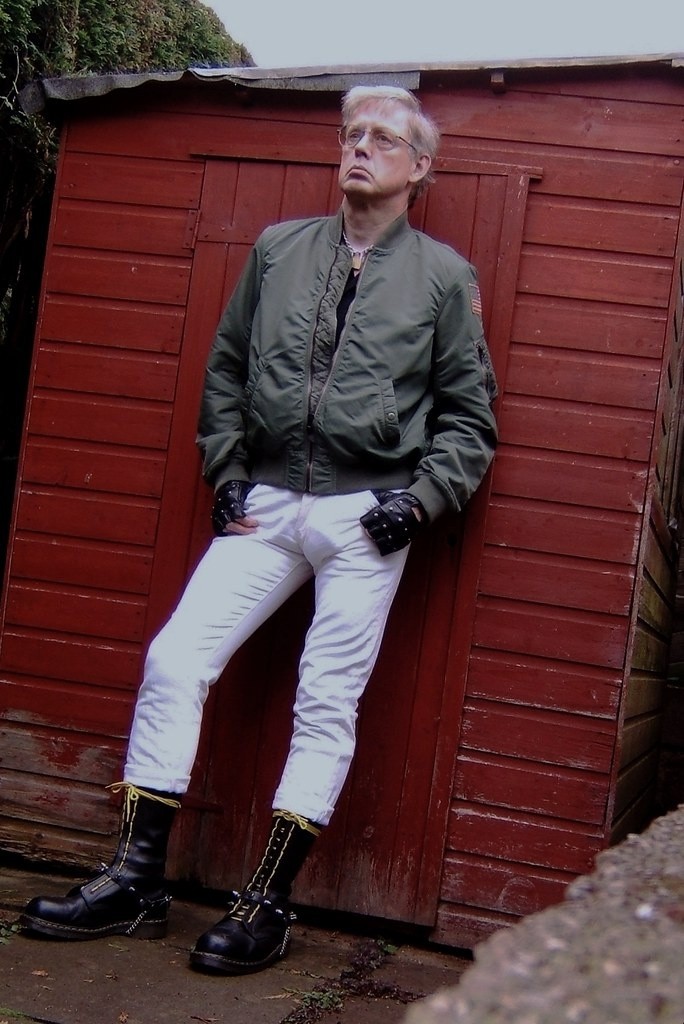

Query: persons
left=22, top=86, right=498, bottom=978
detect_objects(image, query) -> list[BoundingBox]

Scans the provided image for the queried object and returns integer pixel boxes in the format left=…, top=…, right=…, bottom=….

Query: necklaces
left=342, top=230, right=373, bottom=260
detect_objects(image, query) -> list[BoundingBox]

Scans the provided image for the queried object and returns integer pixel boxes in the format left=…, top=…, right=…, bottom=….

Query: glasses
left=337, top=125, right=418, bottom=155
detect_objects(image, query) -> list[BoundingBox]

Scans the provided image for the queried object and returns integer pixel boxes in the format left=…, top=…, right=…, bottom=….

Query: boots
left=17, top=783, right=182, bottom=939
left=189, top=810, right=323, bottom=974
